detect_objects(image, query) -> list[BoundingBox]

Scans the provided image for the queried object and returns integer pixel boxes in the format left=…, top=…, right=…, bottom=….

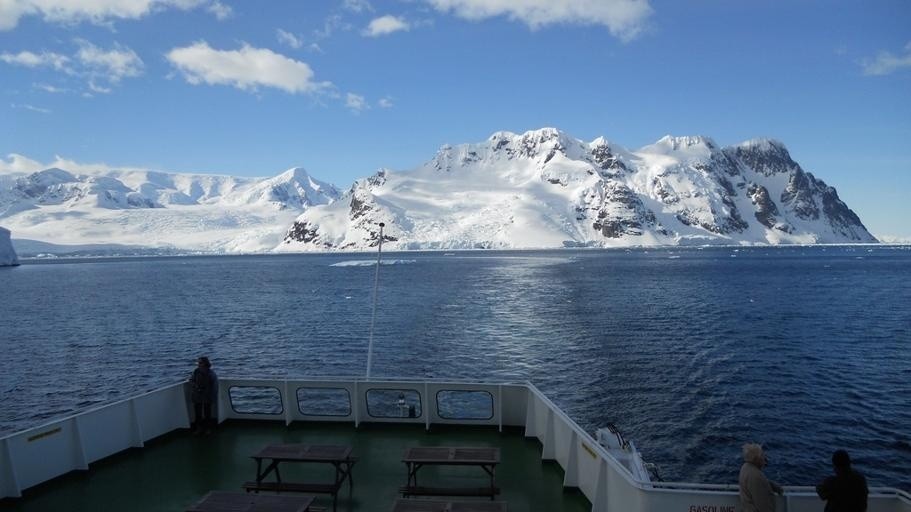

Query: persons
left=814, top=448, right=870, bottom=511
left=188, top=356, right=221, bottom=436
left=736, top=442, right=786, bottom=511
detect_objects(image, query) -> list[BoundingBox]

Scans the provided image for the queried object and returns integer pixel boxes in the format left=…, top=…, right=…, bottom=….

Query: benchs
left=242, top=481, right=338, bottom=501
left=400, top=485, right=500, bottom=503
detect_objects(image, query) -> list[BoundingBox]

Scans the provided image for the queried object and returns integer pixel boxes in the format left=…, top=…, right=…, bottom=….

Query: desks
left=393, top=498, right=509, bottom=512
left=401, top=444, right=502, bottom=487
left=199, top=494, right=317, bottom=511
left=253, top=444, right=354, bottom=495
